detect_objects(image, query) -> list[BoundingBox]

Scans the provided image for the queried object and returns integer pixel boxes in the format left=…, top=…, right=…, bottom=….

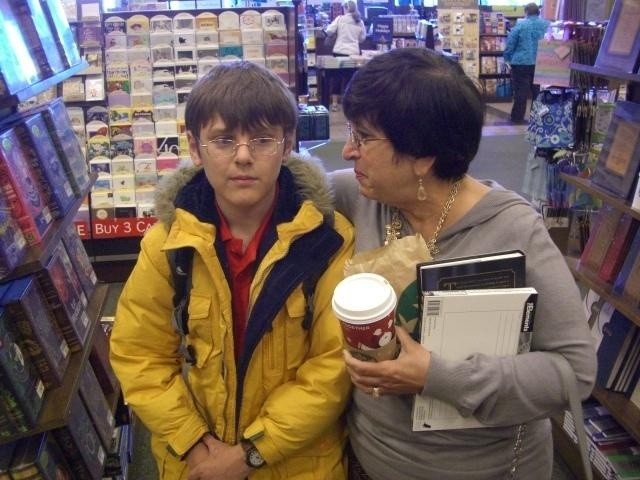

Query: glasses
left=345, top=121, right=393, bottom=149
left=193, top=130, right=289, bottom=159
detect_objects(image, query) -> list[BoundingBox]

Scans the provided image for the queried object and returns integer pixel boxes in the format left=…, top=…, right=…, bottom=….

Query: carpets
left=296, top=134, right=535, bottom=200
left=482, top=103, right=529, bottom=125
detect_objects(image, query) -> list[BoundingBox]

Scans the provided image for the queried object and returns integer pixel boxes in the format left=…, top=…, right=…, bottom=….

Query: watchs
left=240, top=438, right=266, bottom=470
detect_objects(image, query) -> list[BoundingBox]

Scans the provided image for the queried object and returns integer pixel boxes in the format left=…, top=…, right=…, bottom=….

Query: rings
left=370, top=386, right=380, bottom=401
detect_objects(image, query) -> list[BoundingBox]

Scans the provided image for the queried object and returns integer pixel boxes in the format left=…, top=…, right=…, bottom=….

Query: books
left=562, top=402, right=640, bottom=479
left=416, top=249, right=525, bottom=339
left=412, top=288, right=539, bottom=434
left=579, top=291, right=640, bottom=402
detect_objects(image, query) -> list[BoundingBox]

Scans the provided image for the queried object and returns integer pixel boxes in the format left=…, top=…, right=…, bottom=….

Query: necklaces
left=383, top=179, right=461, bottom=255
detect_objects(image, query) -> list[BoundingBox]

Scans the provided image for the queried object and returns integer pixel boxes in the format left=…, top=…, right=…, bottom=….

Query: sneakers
left=331, top=103, right=338, bottom=112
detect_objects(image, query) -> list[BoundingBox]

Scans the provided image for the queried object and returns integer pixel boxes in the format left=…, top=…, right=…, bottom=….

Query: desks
left=313, top=64, right=366, bottom=110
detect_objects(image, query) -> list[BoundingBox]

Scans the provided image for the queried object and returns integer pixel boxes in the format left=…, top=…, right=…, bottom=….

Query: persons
left=504, top=3, right=551, bottom=122
left=326, top=46, right=598, bottom=480
left=324, top=1, right=366, bottom=111
left=109, top=60, right=355, bottom=480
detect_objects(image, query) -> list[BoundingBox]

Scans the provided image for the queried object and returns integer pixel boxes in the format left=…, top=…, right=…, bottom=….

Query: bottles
left=331, top=272, right=400, bottom=363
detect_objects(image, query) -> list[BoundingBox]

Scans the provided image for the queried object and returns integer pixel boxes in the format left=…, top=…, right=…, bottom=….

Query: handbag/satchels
left=533, top=22, right=603, bottom=90
left=529, top=91, right=596, bottom=151
left=495, top=80, right=513, bottom=99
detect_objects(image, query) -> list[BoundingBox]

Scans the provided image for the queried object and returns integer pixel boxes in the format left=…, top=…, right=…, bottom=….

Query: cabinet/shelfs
left=0, top=1, right=139, bottom=479
left=479, top=5, right=511, bottom=102
left=504, top=16, right=523, bottom=31
left=521, top=0, right=640, bottom=480
left=366, top=13, right=439, bottom=52
